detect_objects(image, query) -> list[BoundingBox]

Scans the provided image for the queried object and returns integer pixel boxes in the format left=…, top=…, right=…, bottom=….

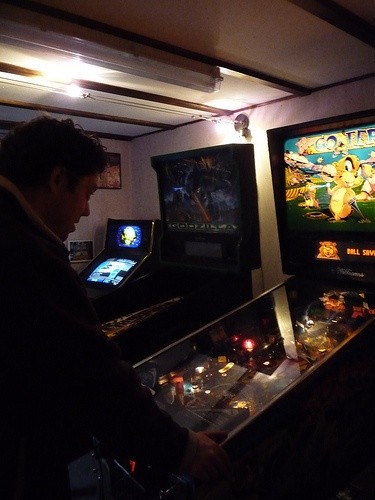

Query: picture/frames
left=67, top=239, right=95, bottom=264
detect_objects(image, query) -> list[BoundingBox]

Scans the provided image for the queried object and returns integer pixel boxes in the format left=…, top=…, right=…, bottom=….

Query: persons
left=0, top=115, right=233, bottom=500
